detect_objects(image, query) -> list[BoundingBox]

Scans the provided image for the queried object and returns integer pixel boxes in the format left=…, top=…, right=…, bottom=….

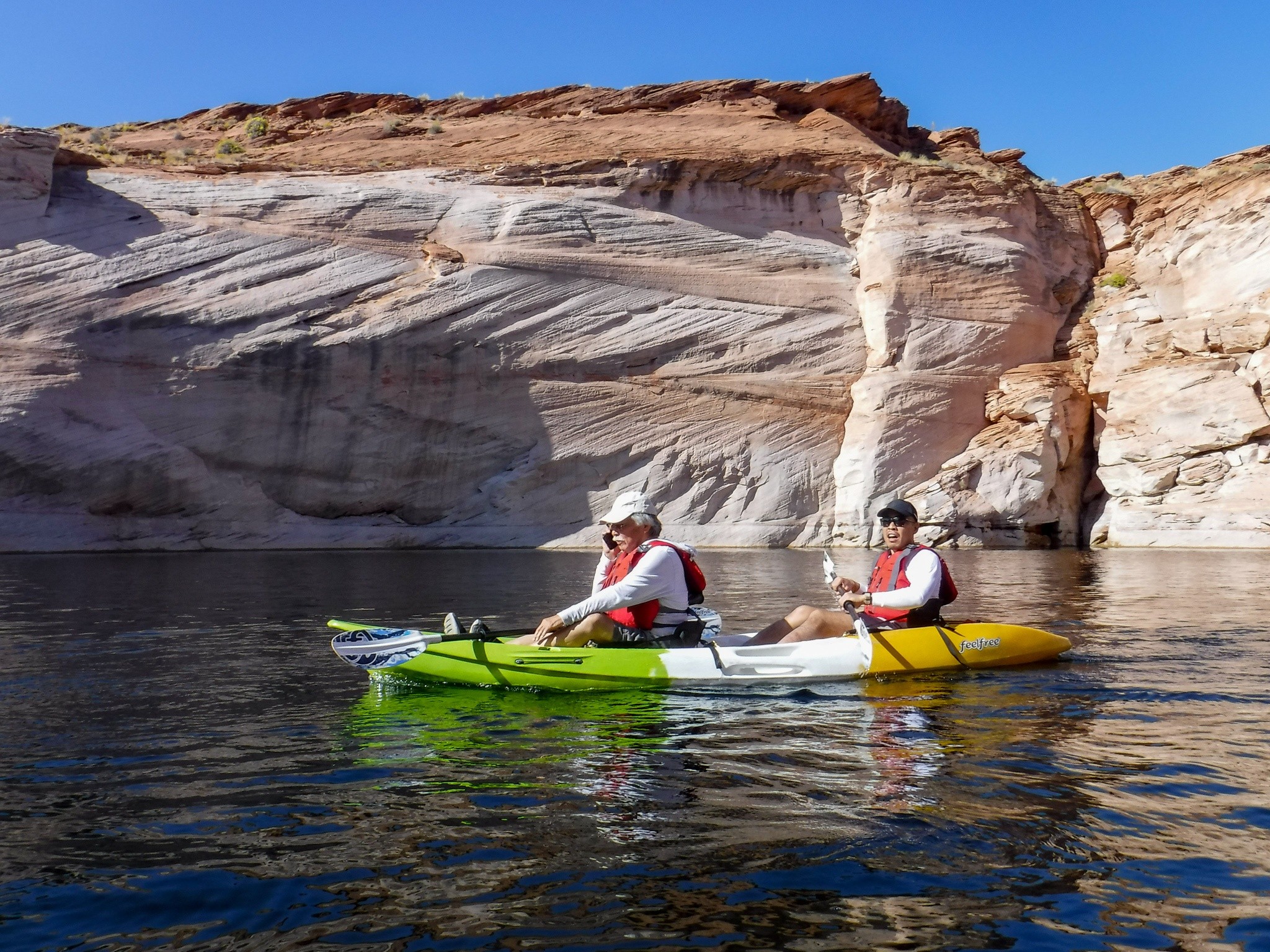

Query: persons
left=739, top=499, right=942, bottom=647
left=443, top=491, right=689, bottom=648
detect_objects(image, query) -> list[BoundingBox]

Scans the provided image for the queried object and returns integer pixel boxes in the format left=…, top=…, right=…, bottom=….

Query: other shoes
left=443, top=612, right=467, bottom=635
left=469, top=619, right=503, bottom=643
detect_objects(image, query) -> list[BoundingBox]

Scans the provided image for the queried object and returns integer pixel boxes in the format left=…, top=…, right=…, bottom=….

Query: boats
left=326, top=616, right=1075, bottom=691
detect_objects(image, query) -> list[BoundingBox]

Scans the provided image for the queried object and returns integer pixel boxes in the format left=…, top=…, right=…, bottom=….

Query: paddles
left=330, top=605, right=722, bottom=670
left=821, top=548, right=875, bottom=671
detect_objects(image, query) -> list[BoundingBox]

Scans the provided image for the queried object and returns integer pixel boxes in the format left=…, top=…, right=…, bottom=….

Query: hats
left=877, top=499, right=918, bottom=522
left=598, top=491, right=657, bottom=525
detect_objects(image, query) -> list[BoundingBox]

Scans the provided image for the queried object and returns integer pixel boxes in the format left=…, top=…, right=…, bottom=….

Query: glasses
left=880, top=516, right=916, bottom=527
left=606, top=521, right=641, bottom=532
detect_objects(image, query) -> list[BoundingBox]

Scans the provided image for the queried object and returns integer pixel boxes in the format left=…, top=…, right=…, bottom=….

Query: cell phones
left=603, top=532, right=618, bottom=549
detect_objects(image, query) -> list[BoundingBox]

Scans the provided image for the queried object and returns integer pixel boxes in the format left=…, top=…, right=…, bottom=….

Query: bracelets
left=864, top=593, right=871, bottom=605
